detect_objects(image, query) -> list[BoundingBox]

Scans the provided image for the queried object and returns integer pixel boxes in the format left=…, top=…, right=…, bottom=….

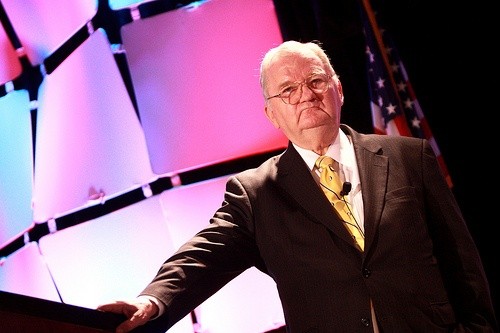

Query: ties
left=316, top=156, right=364, bottom=253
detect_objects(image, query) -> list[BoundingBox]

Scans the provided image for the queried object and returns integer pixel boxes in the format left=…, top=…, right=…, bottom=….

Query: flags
left=361, top=0, right=455, bottom=187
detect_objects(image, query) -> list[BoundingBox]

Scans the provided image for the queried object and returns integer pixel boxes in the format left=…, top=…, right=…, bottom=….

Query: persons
left=95, top=40, right=496, bottom=333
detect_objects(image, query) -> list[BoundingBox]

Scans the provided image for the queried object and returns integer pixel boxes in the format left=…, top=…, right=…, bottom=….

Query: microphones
left=340, top=182, right=351, bottom=197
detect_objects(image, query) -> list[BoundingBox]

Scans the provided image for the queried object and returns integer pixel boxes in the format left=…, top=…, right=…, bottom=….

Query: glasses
left=267, top=73, right=333, bottom=106
